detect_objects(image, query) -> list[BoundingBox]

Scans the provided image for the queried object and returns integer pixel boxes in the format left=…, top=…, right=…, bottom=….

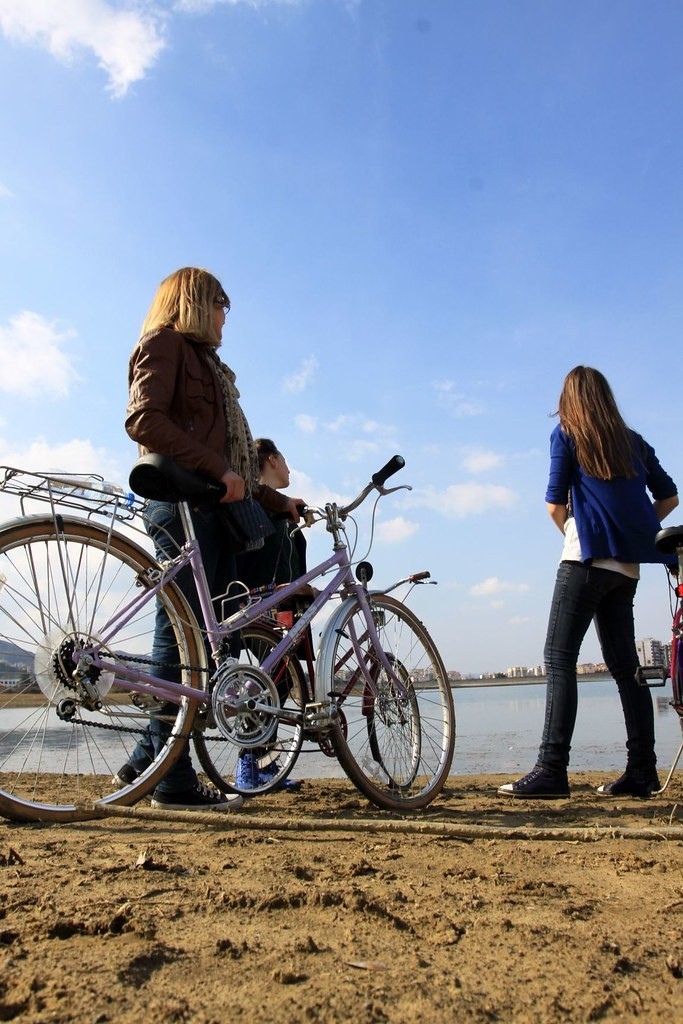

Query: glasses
left=212, top=296, right=230, bottom=315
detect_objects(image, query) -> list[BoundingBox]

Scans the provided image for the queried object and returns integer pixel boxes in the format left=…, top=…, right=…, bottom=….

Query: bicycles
left=0, top=455, right=457, bottom=824
left=634, top=525, right=683, bottom=795
left=194, top=571, right=437, bottom=799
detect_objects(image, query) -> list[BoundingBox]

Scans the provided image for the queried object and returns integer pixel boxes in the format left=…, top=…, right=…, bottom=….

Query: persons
left=496, top=365, right=679, bottom=798
left=110, top=266, right=315, bottom=811
left=234, top=439, right=319, bottom=794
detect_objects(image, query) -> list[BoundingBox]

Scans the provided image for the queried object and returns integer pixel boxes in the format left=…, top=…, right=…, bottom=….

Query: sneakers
left=497, top=767, right=572, bottom=799
left=113, top=763, right=157, bottom=800
left=596, top=772, right=663, bottom=797
left=235, top=753, right=301, bottom=791
left=149, top=779, right=244, bottom=813
